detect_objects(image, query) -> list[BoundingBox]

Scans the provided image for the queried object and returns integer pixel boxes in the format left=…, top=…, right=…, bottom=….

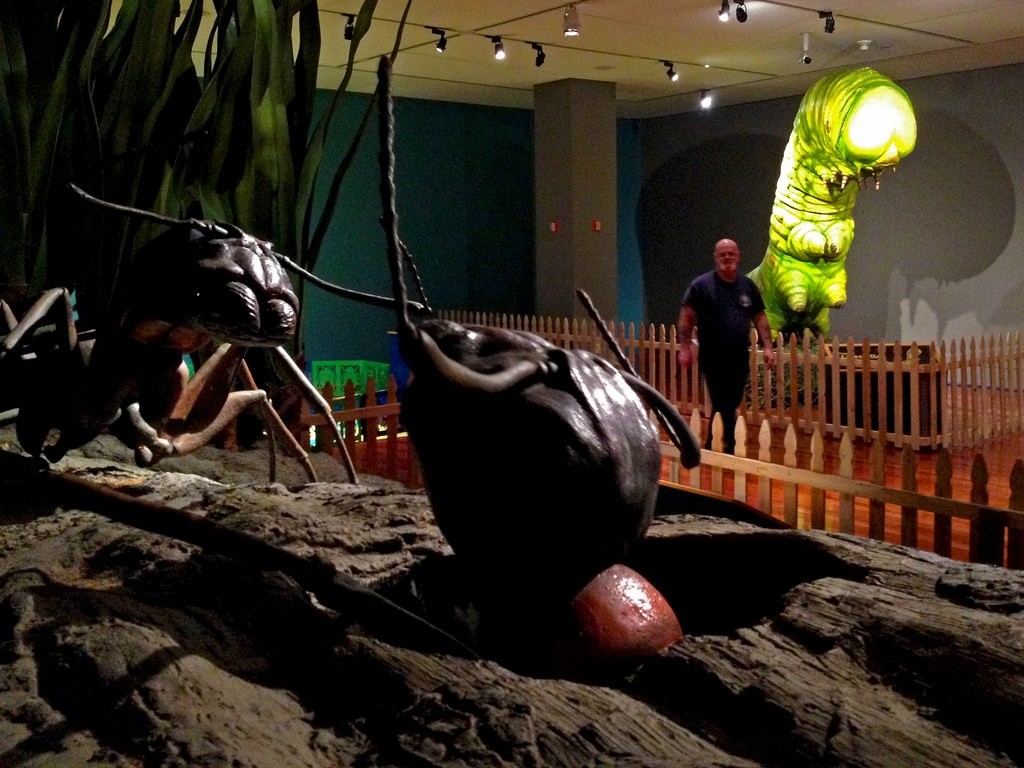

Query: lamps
left=492, top=36, right=506, bottom=60
left=699, top=89, right=711, bottom=108
left=432, top=28, right=447, bottom=52
left=818, top=11, right=835, bottom=34
left=531, top=44, right=546, bottom=68
left=798, top=32, right=812, bottom=65
left=718, top=0, right=730, bottom=22
left=563, top=3, right=580, bottom=38
left=733, top=0, right=747, bottom=23
left=664, top=61, right=678, bottom=82
left=343, top=15, right=354, bottom=40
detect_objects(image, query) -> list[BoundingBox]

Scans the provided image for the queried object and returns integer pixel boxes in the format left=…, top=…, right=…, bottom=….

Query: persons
left=678, top=239, right=775, bottom=456
